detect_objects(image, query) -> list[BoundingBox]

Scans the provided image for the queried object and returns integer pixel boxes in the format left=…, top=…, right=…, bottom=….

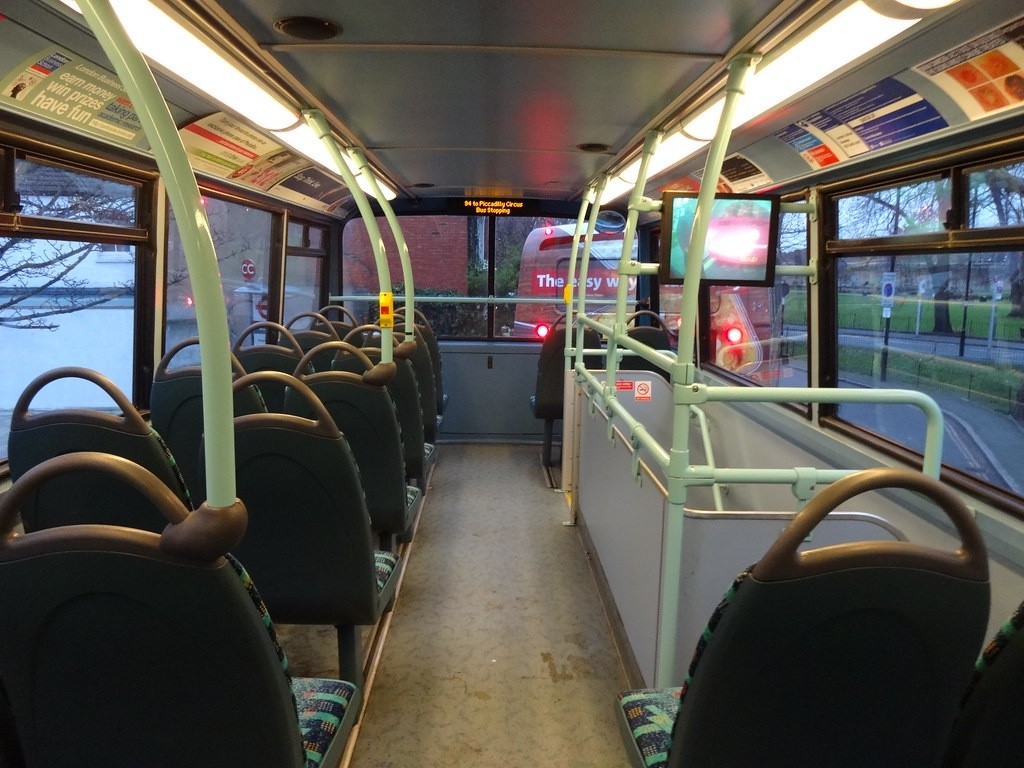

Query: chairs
left=0, top=303, right=1024, bottom=768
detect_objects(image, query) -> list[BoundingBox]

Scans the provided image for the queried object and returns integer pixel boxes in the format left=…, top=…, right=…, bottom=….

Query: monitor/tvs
left=660, top=190, right=781, bottom=288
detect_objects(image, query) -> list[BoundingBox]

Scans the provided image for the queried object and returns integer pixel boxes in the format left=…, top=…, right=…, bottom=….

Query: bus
left=513, top=220, right=725, bottom=356
